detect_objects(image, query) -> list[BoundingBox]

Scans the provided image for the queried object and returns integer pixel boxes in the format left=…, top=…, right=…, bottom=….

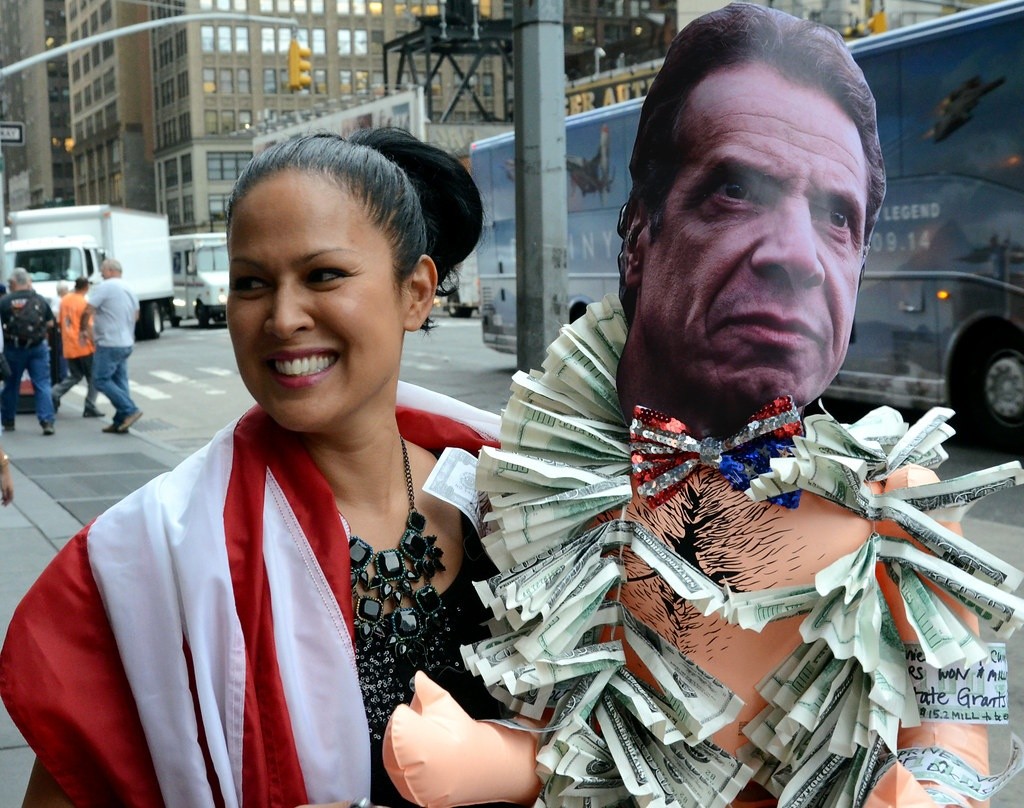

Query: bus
left=468, top=2, right=1024, bottom=457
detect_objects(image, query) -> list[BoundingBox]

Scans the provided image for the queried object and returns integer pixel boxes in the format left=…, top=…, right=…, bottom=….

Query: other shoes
left=53, top=398, right=60, bottom=412
left=4, top=424, right=13, bottom=431
left=41, top=422, right=55, bottom=434
left=103, top=424, right=127, bottom=433
left=118, top=410, right=142, bottom=431
left=84, top=407, right=105, bottom=416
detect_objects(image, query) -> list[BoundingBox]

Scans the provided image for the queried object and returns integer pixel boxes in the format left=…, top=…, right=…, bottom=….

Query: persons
left=380, top=1, right=1024, bottom=808
left=0, top=258, right=144, bottom=506
left=0, top=123, right=532, bottom=808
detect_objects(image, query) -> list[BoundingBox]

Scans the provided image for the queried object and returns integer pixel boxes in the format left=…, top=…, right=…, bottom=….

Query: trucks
left=440, top=249, right=480, bottom=318
left=170, top=232, right=229, bottom=330
left=2, top=204, right=175, bottom=340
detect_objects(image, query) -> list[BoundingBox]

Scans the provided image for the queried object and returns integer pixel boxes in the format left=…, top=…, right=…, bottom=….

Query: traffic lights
left=288, top=41, right=313, bottom=92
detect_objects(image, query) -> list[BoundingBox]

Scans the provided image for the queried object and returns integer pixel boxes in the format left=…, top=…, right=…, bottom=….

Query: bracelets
left=0, top=455, right=9, bottom=469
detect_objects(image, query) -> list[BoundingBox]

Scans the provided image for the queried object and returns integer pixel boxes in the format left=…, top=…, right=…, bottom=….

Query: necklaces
left=348, top=435, right=448, bottom=668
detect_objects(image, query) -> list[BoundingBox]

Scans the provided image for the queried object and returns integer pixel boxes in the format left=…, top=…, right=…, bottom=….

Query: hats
left=75, top=277, right=94, bottom=287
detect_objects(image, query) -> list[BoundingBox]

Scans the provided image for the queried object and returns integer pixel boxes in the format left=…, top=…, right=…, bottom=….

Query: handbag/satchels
left=0, top=353, right=11, bottom=379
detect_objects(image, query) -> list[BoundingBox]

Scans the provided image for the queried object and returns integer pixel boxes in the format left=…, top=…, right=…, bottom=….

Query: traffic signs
left=1, top=122, right=24, bottom=145
